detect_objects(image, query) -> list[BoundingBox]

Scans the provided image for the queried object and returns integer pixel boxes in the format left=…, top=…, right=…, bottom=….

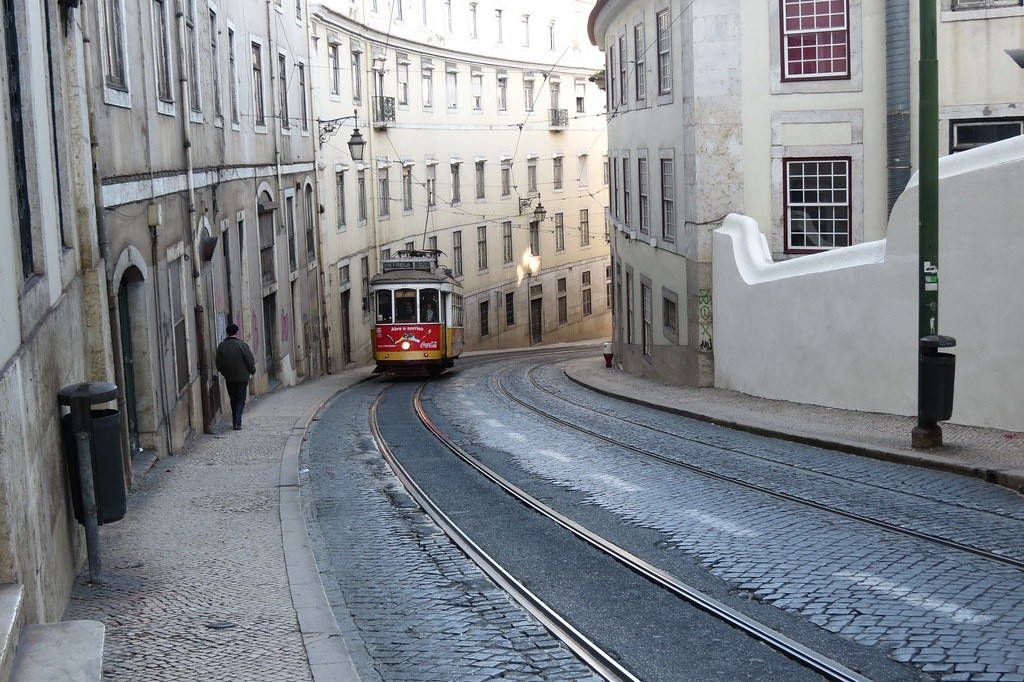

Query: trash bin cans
left=918, top=335, right=957, bottom=420
left=57, top=381, right=129, bottom=529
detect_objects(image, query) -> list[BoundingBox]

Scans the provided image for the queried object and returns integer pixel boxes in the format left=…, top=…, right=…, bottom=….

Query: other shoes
left=232, top=421, right=241, bottom=430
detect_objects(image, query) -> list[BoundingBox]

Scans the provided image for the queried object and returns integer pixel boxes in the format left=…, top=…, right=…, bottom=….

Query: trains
left=369, top=192, right=463, bottom=374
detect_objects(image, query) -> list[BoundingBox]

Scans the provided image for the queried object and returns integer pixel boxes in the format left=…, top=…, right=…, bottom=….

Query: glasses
left=406, top=305, right=412, bottom=307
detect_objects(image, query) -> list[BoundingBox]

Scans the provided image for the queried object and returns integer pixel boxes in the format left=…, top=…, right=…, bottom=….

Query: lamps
left=318, top=110, right=368, bottom=160
left=518, top=192, right=547, bottom=222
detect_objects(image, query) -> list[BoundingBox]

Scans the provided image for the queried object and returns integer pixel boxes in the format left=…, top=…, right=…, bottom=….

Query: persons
left=215, top=323, right=257, bottom=430
left=402, top=297, right=438, bottom=321
